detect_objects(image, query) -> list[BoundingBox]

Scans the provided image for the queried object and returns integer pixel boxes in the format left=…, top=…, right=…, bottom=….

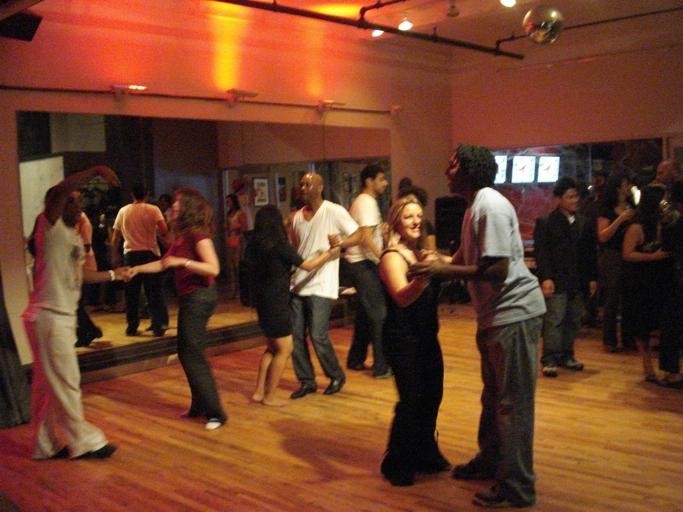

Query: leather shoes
left=289, top=380, right=317, bottom=399
left=76, top=328, right=103, bottom=346
left=325, top=376, right=347, bottom=396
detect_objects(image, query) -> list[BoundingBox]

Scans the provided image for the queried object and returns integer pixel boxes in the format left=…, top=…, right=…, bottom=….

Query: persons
left=622, top=182, right=673, bottom=382
left=158, top=193, right=176, bottom=298
left=398, top=187, right=436, bottom=252
left=595, top=168, right=636, bottom=351
left=378, top=193, right=452, bottom=488
left=111, top=181, right=168, bottom=336
left=69, top=210, right=103, bottom=347
left=406, top=143, right=547, bottom=508
left=287, top=172, right=363, bottom=400
left=126, top=186, right=228, bottom=430
left=657, top=179, right=683, bottom=388
left=581, top=169, right=609, bottom=217
left=244, top=203, right=341, bottom=407
left=20, top=164, right=130, bottom=461
left=343, top=164, right=391, bottom=379
left=221, top=193, right=247, bottom=300
left=104, top=205, right=124, bottom=313
left=656, top=159, right=681, bottom=202
left=533, top=175, right=599, bottom=377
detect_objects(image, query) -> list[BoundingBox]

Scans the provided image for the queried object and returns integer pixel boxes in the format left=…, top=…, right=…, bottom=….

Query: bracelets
left=108, top=270, right=115, bottom=281
left=184, top=259, right=191, bottom=267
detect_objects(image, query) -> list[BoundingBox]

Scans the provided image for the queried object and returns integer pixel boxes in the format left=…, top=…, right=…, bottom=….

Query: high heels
left=661, top=374, right=683, bottom=389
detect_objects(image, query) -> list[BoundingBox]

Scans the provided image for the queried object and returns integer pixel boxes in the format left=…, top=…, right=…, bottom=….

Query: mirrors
left=481, top=138, right=664, bottom=254
left=15, top=109, right=392, bottom=355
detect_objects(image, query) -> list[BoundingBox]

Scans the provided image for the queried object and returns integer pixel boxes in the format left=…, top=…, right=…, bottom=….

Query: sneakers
left=453, top=459, right=495, bottom=478
left=565, top=358, right=583, bottom=370
left=472, top=485, right=529, bottom=507
left=372, top=366, right=392, bottom=378
left=545, top=364, right=556, bottom=377
